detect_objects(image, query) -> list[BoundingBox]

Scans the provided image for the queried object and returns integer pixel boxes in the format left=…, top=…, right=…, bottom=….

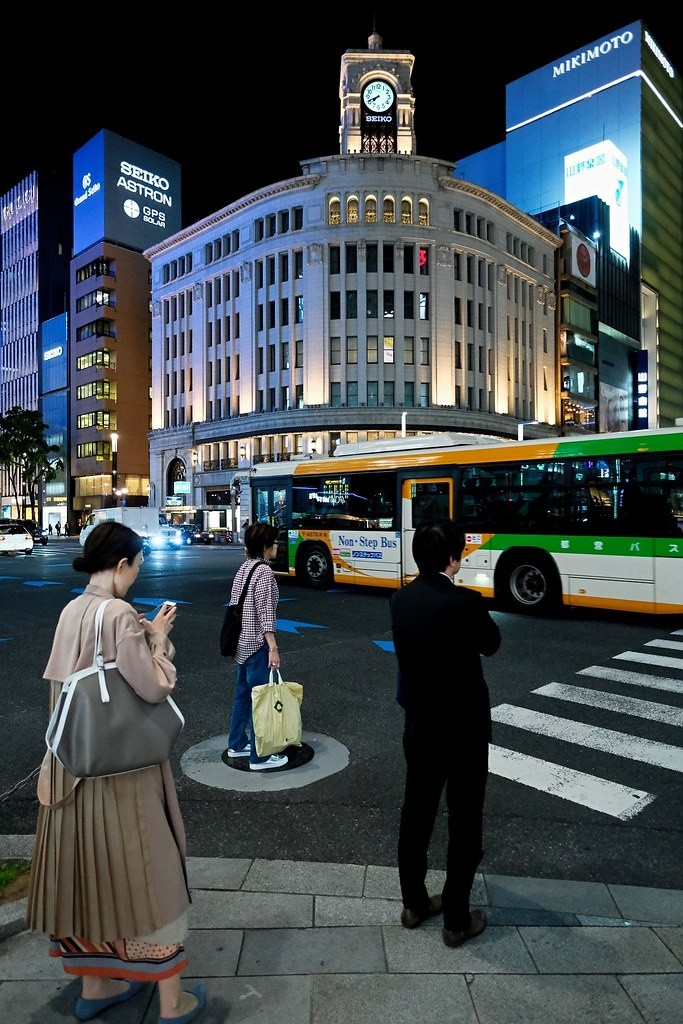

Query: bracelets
left=269, top=647, right=278, bottom=651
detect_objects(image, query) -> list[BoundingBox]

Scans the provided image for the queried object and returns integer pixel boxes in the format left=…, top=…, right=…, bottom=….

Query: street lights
left=110, top=433, right=119, bottom=507
left=116, top=488, right=128, bottom=507
left=517, top=424, right=525, bottom=442
left=146, top=482, right=155, bottom=508
left=400, top=412, right=408, bottom=437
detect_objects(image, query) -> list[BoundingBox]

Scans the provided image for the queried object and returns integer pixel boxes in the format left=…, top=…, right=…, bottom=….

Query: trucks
left=78, top=506, right=160, bottom=556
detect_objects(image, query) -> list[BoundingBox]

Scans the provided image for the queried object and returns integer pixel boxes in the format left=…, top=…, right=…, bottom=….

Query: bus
left=230, top=424, right=683, bottom=625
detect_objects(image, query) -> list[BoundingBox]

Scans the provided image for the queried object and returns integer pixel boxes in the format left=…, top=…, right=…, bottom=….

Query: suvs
left=150, top=524, right=182, bottom=551
left=171, top=524, right=215, bottom=544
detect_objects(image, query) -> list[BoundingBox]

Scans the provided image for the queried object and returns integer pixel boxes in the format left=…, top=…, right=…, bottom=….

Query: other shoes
left=76, top=977, right=143, bottom=1020
left=158, top=983, right=207, bottom=1024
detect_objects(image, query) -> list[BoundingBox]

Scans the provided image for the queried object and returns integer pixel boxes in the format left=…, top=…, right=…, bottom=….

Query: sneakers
left=250, top=753, right=288, bottom=770
left=228, top=744, right=251, bottom=757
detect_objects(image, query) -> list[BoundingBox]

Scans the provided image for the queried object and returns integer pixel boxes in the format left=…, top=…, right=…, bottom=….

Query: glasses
left=274, top=542, right=280, bottom=545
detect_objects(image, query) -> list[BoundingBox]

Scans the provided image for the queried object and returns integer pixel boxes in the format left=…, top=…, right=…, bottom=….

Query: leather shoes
left=401, top=894, right=441, bottom=929
left=442, top=909, right=487, bottom=947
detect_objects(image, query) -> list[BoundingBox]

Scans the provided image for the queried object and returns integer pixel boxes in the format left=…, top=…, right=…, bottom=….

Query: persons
left=49, top=521, right=70, bottom=537
left=227, top=522, right=289, bottom=771
left=245, top=519, right=249, bottom=531
left=24, top=521, right=207, bottom=1024
left=390, top=514, right=502, bottom=948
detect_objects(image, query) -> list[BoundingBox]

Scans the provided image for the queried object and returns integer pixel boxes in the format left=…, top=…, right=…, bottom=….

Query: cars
left=0, top=524, right=34, bottom=555
left=0, top=518, right=50, bottom=546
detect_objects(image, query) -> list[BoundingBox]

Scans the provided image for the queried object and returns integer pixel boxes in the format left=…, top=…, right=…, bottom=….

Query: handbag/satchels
left=219, top=604, right=243, bottom=658
left=251, top=668, right=304, bottom=757
left=45, top=598, right=185, bottom=780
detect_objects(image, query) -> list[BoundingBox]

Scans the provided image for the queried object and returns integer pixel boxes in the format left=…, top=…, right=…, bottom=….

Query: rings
left=273, top=663, right=276, bottom=665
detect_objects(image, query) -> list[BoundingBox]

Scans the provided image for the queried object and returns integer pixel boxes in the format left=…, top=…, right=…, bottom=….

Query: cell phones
left=139, top=601, right=177, bottom=623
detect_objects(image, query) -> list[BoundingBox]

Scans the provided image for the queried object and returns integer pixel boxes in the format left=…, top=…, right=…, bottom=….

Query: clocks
left=361, top=78, right=395, bottom=113
